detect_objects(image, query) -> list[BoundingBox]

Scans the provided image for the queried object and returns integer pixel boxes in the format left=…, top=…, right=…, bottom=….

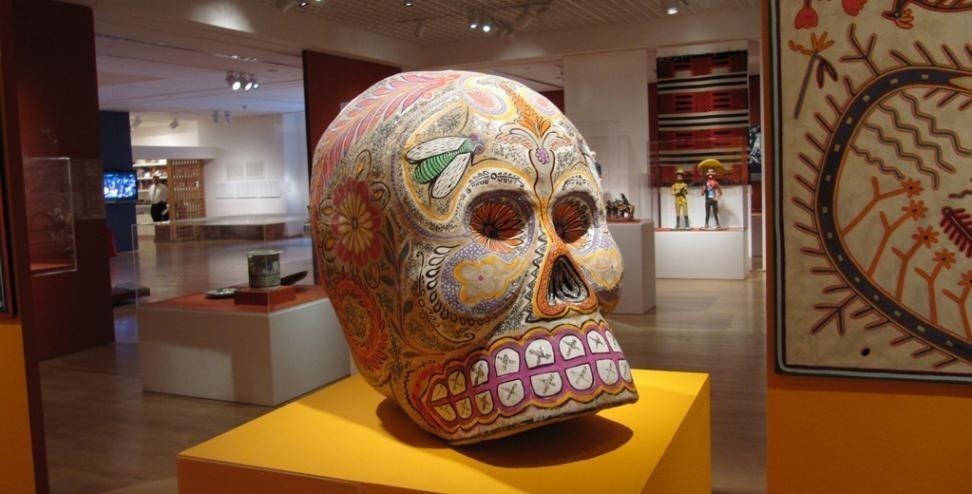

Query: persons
left=697, top=159, right=725, bottom=229
left=671, top=168, right=689, bottom=228
left=148, top=176, right=170, bottom=222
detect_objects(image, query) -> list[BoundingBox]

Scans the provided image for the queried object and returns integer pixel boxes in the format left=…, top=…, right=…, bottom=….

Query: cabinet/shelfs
left=135, top=158, right=201, bottom=213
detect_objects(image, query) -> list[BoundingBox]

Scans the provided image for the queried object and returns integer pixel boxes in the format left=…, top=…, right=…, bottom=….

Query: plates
left=205, top=287, right=236, bottom=298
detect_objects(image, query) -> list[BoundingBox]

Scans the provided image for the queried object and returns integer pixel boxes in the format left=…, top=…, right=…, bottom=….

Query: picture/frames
left=759, top=0, right=972, bottom=399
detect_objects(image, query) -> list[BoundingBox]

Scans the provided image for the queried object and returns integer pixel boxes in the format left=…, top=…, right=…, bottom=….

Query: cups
left=242, top=248, right=284, bottom=289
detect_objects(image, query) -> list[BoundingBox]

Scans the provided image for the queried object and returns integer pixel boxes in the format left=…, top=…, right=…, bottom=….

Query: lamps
left=460, top=9, right=535, bottom=42
left=224, top=69, right=262, bottom=92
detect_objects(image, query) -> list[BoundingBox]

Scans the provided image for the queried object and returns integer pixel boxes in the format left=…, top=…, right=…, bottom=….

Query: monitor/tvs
left=103, top=168, right=137, bottom=205
